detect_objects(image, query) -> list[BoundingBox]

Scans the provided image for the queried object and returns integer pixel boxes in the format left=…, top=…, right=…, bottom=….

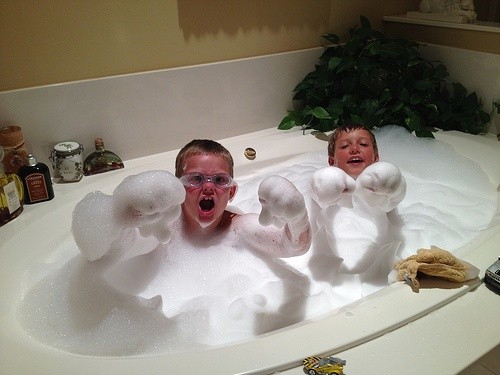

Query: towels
left=397, top=243, right=479, bottom=288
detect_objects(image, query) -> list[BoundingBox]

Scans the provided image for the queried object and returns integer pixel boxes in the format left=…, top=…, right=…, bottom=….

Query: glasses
left=180, top=174, right=232, bottom=189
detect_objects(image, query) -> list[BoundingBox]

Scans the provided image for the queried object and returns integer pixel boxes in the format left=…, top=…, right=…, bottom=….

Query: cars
left=484, top=255, right=500, bottom=293
left=303, top=355, right=347, bottom=375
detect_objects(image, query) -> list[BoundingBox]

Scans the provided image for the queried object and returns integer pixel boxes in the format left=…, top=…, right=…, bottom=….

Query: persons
left=310, top=122, right=406, bottom=206
left=70, top=139, right=311, bottom=257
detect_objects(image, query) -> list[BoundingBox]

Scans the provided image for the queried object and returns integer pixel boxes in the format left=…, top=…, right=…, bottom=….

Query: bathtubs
left=13, top=113, right=497, bottom=358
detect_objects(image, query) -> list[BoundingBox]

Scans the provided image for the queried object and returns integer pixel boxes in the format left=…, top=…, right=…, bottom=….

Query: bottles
left=17, top=153, right=54, bottom=204
left=0, top=145, right=24, bottom=226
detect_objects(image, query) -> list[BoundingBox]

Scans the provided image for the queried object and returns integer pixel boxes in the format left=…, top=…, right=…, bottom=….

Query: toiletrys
left=84, top=137, right=125, bottom=175
left=0, top=125, right=56, bottom=226
left=50, top=141, right=83, bottom=184
